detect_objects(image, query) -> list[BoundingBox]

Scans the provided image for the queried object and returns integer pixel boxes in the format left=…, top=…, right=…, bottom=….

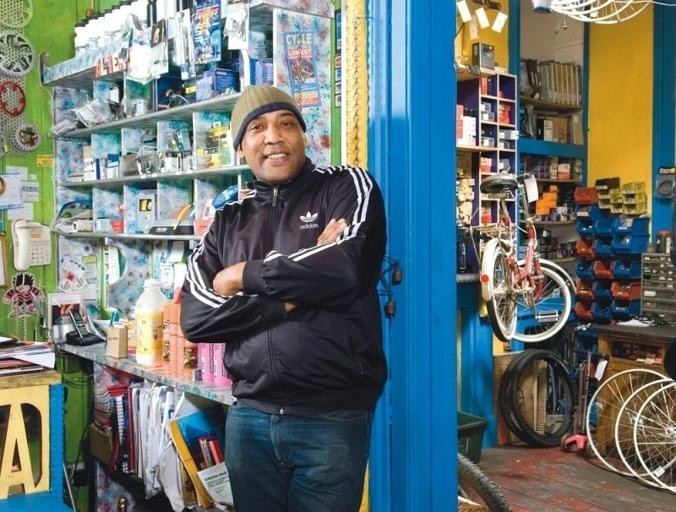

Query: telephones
left=66, top=309, right=99, bottom=345
left=11, top=221, right=52, bottom=272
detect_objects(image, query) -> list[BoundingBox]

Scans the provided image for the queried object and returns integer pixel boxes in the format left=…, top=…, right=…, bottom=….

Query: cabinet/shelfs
left=3, top=4, right=332, bottom=511
left=456, top=62, right=586, bottom=441
left=640, top=253, right=676, bottom=325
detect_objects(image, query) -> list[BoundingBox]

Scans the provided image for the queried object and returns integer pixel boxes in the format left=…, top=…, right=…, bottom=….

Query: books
left=0, top=329, right=55, bottom=378
left=108, top=378, right=225, bottom=510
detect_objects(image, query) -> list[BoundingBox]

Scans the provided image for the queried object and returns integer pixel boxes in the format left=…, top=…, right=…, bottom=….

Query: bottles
left=135, top=279, right=164, bottom=367
left=656, top=231, right=672, bottom=253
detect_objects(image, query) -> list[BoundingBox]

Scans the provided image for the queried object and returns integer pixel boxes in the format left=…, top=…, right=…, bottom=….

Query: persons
left=180, top=84, right=387, bottom=512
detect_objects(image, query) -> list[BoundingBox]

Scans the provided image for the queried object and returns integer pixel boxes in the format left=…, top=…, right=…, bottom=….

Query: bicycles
left=469, top=172, right=577, bottom=344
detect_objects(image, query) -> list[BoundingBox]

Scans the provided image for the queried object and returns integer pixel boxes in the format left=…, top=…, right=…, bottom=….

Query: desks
left=593, top=325, right=676, bottom=459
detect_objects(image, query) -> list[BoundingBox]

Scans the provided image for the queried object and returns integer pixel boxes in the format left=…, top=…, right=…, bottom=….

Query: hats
left=232, top=86, right=306, bottom=151
